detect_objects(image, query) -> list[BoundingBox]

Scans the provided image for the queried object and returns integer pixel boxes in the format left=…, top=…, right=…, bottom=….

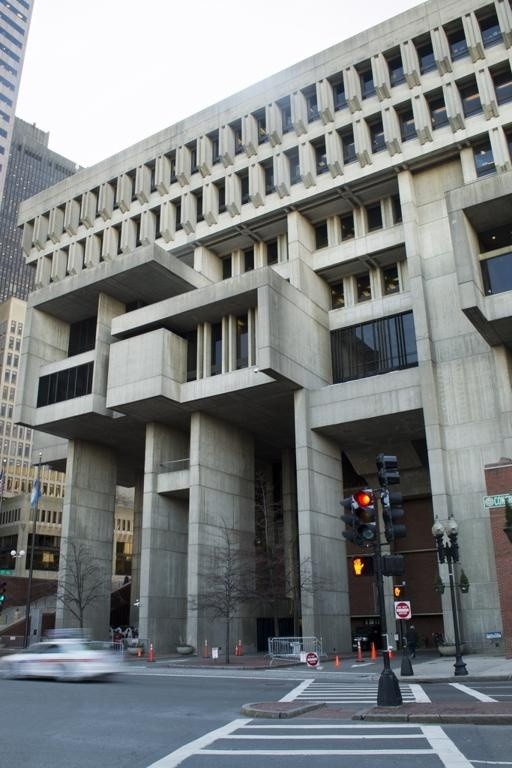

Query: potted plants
left=176, top=643, right=193, bottom=654
left=127, top=642, right=143, bottom=654
left=438, top=640, right=466, bottom=656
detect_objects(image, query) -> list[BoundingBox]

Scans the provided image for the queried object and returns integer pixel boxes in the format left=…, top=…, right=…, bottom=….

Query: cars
left=1, top=640, right=121, bottom=684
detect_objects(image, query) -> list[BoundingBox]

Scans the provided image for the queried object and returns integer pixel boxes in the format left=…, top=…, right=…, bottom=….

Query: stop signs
left=394, top=600, right=412, bottom=620
left=306, top=651, right=320, bottom=667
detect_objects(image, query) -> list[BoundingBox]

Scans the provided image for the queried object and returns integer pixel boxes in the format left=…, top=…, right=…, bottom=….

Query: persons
left=405, top=624, right=419, bottom=658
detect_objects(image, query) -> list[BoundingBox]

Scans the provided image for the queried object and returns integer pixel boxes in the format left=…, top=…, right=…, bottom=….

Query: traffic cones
left=236, top=638, right=243, bottom=654
left=335, top=655, right=341, bottom=668
left=138, top=648, right=142, bottom=656
left=203, top=634, right=210, bottom=659
left=147, top=639, right=156, bottom=662
left=388, top=646, right=395, bottom=659
left=370, top=642, right=377, bottom=659
left=354, top=638, right=364, bottom=662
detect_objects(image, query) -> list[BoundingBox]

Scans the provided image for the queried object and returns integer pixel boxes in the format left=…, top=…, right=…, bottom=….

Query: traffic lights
left=340, top=494, right=362, bottom=545
left=377, top=451, right=401, bottom=486
left=353, top=486, right=379, bottom=546
left=393, top=587, right=404, bottom=598
left=0, top=582, right=6, bottom=606
left=352, top=554, right=375, bottom=579
left=380, top=490, right=407, bottom=543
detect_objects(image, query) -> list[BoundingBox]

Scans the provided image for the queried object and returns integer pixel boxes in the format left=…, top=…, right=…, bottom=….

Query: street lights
left=432, top=514, right=471, bottom=676
left=9, top=549, right=25, bottom=575
